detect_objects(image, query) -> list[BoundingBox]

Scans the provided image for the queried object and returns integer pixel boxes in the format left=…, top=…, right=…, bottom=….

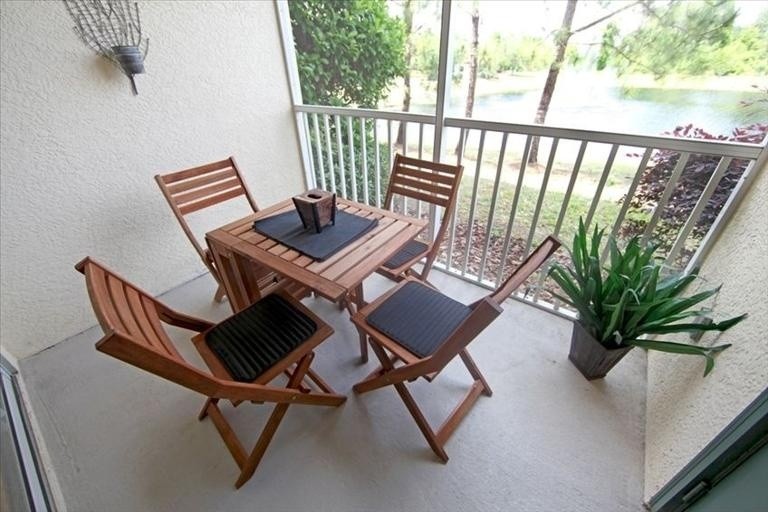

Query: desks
left=205, top=188, right=429, bottom=363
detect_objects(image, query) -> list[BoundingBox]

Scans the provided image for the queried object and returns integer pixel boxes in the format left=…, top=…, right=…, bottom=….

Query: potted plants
left=548, top=216, right=750, bottom=380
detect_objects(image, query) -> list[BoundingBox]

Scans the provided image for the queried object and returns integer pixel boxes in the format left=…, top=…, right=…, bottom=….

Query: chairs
left=371, top=154, right=464, bottom=292
left=75, top=256, right=347, bottom=488
left=349, top=231, right=562, bottom=462
left=154, top=157, right=319, bottom=303
